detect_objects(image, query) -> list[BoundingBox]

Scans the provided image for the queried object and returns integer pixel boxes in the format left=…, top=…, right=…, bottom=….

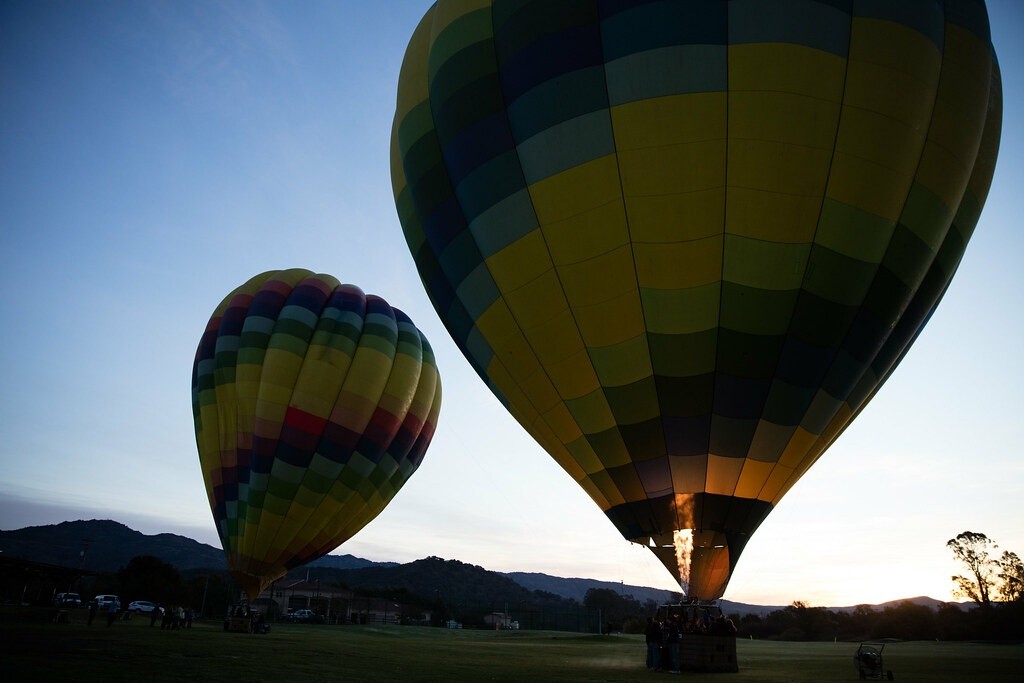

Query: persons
left=643, top=607, right=737, bottom=674
left=87, top=598, right=99, bottom=625
left=106, top=597, right=118, bottom=628
left=149, top=606, right=194, bottom=631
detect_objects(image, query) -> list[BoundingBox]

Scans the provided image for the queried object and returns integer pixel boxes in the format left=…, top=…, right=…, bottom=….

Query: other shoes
left=677, top=670, right=681, bottom=674
left=668, top=670, right=677, bottom=674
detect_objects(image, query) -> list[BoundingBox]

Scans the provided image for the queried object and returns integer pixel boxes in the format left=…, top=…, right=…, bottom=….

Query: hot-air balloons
left=389, top=0, right=1005, bottom=674
left=191, top=268, right=443, bottom=635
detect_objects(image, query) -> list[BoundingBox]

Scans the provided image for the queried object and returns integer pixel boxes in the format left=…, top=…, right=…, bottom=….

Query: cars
left=128, top=600, right=165, bottom=616
left=287, top=609, right=325, bottom=623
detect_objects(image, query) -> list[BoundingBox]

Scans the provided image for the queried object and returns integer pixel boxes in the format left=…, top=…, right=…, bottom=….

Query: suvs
left=88, top=595, right=120, bottom=612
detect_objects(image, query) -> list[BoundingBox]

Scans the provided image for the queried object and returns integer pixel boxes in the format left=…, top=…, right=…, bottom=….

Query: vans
left=54, top=593, right=82, bottom=608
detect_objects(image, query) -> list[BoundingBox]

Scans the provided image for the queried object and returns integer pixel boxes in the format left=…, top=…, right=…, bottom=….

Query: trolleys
left=853, top=642, right=895, bottom=681
